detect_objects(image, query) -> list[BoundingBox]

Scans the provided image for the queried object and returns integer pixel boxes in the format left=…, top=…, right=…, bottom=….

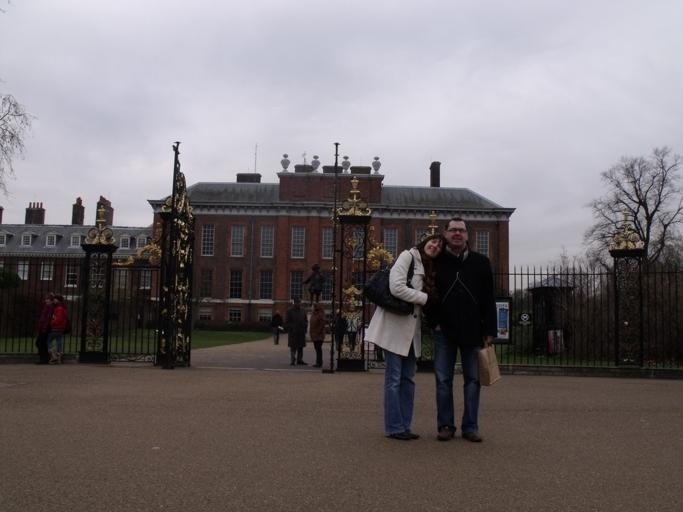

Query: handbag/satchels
left=362, top=252, right=415, bottom=316
left=477, top=343, right=501, bottom=387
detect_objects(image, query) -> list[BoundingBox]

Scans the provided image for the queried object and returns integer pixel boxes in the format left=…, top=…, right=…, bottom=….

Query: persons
left=272, top=310, right=283, bottom=345
left=423, top=218, right=497, bottom=440
left=364, top=234, right=446, bottom=440
left=34, top=292, right=55, bottom=365
left=47, top=294, right=67, bottom=363
left=335, top=308, right=357, bottom=353
left=303, top=264, right=325, bottom=306
left=310, top=302, right=326, bottom=368
left=285, top=296, right=308, bottom=365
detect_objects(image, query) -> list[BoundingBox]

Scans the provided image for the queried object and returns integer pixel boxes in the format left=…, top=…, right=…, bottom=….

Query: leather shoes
left=409, top=434, right=419, bottom=440
left=387, top=432, right=412, bottom=440
left=462, top=432, right=482, bottom=442
left=437, top=427, right=454, bottom=441
